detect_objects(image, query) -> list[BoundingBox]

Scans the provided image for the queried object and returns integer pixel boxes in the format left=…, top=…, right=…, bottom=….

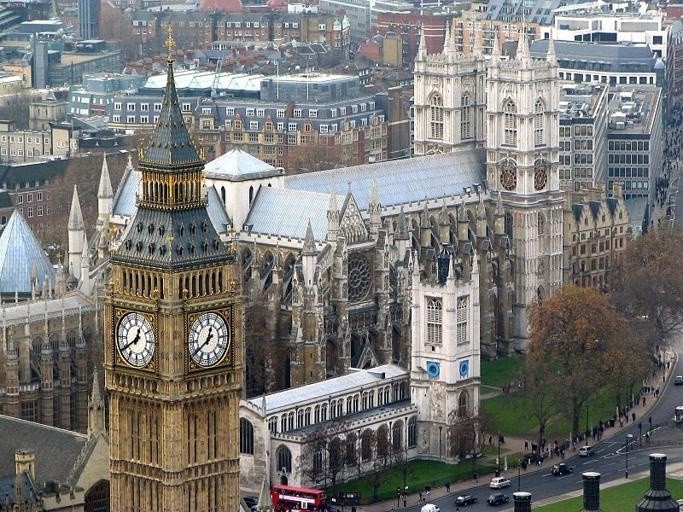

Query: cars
left=421, top=504, right=440, bottom=512
left=675, top=376, right=683, bottom=385
left=487, top=493, right=509, bottom=506
left=551, top=463, right=573, bottom=476
left=489, top=476, right=512, bottom=489
left=455, top=494, right=478, bottom=507
left=579, top=446, right=594, bottom=457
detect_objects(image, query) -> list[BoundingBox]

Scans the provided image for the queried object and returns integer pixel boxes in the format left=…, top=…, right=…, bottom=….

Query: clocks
left=112, top=304, right=159, bottom=373
left=184, top=306, right=235, bottom=375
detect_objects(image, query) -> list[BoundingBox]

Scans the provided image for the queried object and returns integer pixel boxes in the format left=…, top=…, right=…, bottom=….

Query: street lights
left=626, top=434, right=633, bottom=478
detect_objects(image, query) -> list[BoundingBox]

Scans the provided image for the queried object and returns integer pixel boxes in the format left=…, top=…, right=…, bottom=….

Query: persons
left=421, top=497, right=426, bottom=505
left=398, top=485, right=433, bottom=502
left=455, top=508, right=460, bottom=512
left=488, top=436, right=492, bottom=446
left=521, top=342, right=676, bottom=470
left=472, top=471, right=478, bottom=482
left=444, top=481, right=450, bottom=492
left=648, top=100, right=682, bottom=230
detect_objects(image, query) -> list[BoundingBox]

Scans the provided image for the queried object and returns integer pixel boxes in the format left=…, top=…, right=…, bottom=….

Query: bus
left=271, top=484, right=326, bottom=508
left=673, top=406, right=683, bottom=425
